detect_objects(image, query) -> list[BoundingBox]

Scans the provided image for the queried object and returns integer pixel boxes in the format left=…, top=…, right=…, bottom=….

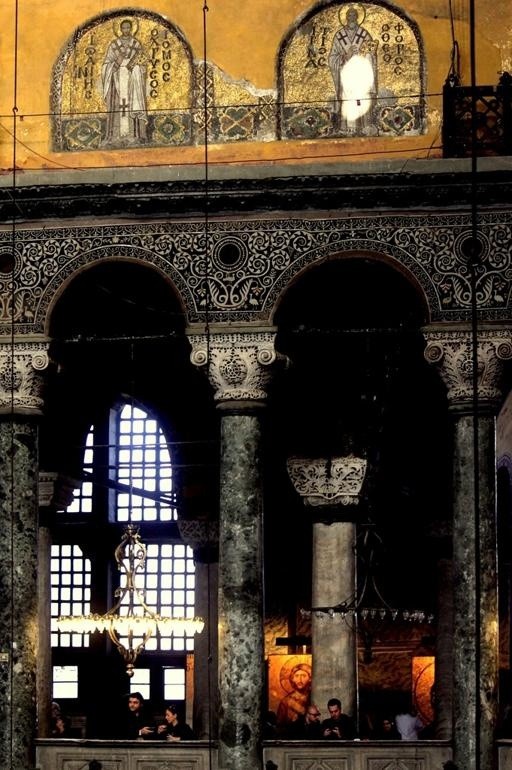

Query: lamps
left=298, top=330, right=435, bottom=666
left=55, top=336, right=206, bottom=676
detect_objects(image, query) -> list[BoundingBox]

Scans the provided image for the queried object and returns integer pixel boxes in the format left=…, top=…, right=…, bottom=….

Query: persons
left=51, top=701, right=71, bottom=738
left=321, top=699, right=356, bottom=740
left=265, top=710, right=279, bottom=739
left=155, top=703, right=197, bottom=740
left=119, top=693, right=155, bottom=740
left=295, top=706, right=321, bottom=739
left=101, top=19, right=151, bottom=147
left=325, top=5, right=382, bottom=138
left=394, top=699, right=426, bottom=740
left=277, top=663, right=312, bottom=724
left=371, top=716, right=401, bottom=740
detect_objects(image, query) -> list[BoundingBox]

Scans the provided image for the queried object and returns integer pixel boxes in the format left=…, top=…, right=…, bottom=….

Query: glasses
left=307, top=713, right=321, bottom=718
